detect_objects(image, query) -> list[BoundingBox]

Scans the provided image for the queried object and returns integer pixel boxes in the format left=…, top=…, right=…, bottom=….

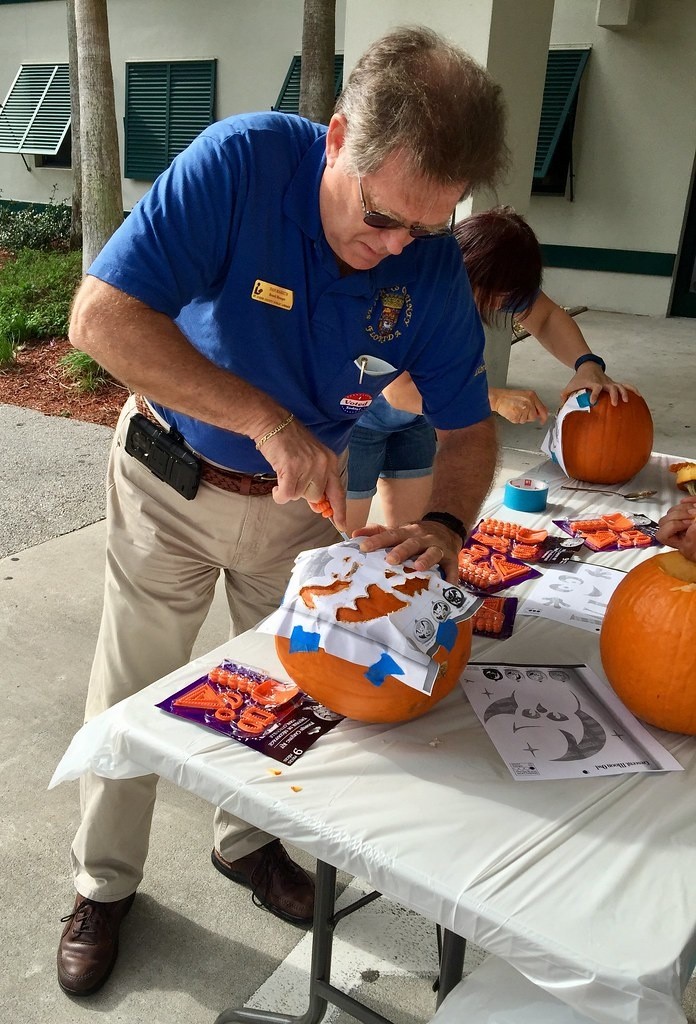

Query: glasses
left=355, top=160, right=456, bottom=240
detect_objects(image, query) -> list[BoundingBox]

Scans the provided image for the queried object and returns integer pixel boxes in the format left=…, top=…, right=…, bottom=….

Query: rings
left=427, top=545, right=444, bottom=558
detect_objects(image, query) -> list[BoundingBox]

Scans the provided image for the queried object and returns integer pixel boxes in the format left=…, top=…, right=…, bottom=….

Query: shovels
left=570, top=512, right=633, bottom=531
left=207, top=668, right=300, bottom=707
left=480, top=519, right=549, bottom=543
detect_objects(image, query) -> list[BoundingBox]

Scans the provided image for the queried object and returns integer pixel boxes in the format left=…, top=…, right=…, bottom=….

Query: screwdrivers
left=307, top=493, right=351, bottom=542
left=538, top=409, right=560, bottom=417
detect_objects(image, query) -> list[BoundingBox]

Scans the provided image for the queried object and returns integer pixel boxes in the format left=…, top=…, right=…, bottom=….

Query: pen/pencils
left=358, top=357, right=370, bottom=384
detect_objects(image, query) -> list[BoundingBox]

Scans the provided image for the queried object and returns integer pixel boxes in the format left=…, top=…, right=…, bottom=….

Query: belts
left=134, top=392, right=281, bottom=496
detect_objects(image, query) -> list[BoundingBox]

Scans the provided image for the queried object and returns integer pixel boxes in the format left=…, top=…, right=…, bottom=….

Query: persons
left=655, top=496, right=696, bottom=562
left=346, top=205, right=641, bottom=540
left=57, top=29, right=501, bottom=995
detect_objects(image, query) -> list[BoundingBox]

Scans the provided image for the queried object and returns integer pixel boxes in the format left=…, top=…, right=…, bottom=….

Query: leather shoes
left=211, top=839, right=318, bottom=922
left=57, top=892, right=135, bottom=997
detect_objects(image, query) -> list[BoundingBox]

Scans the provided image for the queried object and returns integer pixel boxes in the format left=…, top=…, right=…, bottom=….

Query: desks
left=47, top=452, right=696, bottom=1024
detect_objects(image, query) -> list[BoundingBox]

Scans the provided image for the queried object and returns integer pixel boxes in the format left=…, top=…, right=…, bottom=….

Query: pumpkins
left=560, top=388, right=653, bottom=484
left=600, top=550, right=696, bottom=736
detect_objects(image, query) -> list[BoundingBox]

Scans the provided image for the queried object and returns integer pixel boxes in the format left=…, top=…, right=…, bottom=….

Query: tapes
left=502, top=477, right=549, bottom=512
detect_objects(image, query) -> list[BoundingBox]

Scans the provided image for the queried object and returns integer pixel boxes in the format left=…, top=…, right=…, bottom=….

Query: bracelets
left=256, top=414, right=294, bottom=450
left=575, top=354, right=606, bottom=372
left=421, top=512, right=468, bottom=549
